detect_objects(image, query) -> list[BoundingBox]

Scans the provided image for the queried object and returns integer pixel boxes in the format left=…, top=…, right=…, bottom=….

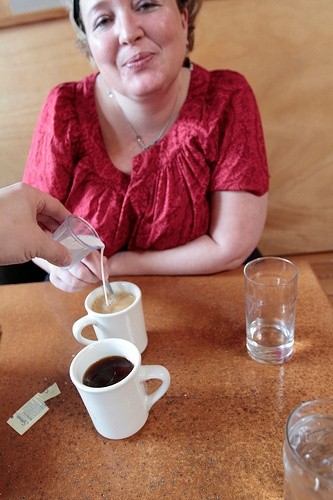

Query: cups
left=69, top=338, right=170, bottom=439
left=241, top=257, right=298, bottom=365
left=281, top=399, right=333, bottom=500
left=52, top=214, right=103, bottom=270
left=71, top=280, right=148, bottom=356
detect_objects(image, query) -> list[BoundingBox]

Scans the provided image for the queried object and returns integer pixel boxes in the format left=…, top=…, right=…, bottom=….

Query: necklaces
left=107, top=78, right=181, bottom=150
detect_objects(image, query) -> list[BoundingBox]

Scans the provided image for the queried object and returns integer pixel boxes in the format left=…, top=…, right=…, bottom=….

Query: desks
left=0, top=259, right=332, bottom=500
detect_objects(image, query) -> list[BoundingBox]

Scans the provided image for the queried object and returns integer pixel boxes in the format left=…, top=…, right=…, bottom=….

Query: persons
left=0, top=182, right=80, bottom=267
left=22, top=0, right=271, bottom=292
